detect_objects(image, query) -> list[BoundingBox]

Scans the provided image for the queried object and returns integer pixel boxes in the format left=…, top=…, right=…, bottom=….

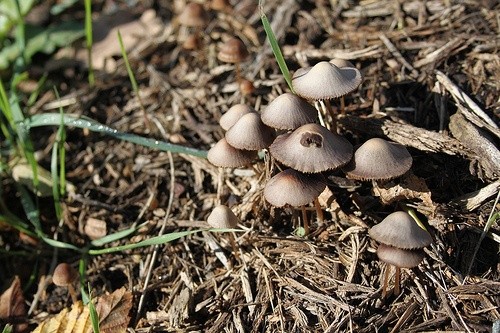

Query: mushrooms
left=52, top=262, right=79, bottom=297
left=206, top=57, right=433, bottom=268
